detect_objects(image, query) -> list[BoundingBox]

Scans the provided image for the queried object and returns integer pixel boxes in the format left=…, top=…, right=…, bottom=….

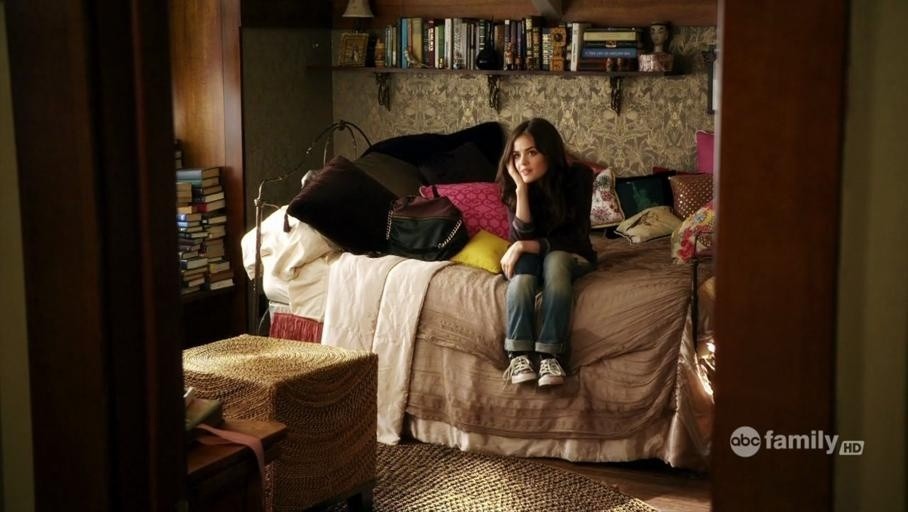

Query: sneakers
left=510, top=351, right=538, bottom=384
left=538, top=353, right=565, bottom=388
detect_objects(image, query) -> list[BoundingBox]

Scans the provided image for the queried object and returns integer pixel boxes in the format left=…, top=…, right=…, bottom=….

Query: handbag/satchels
left=385, top=185, right=469, bottom=262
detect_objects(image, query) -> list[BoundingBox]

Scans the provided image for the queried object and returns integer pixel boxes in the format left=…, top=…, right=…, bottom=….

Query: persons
left=495, top=117, right=599, bottom=387
left=648, top=15, right=674, bottom=53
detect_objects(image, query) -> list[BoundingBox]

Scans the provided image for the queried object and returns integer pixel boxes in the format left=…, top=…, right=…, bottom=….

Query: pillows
left=588, top=167, right=719, bottom=263
left=418, top=181, right=529, bottom=243
left=450, top=229, right=517, bottom=274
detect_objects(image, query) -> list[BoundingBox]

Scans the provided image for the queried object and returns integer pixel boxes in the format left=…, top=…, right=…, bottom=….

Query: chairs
left=270, top=312, right=325, bottom=344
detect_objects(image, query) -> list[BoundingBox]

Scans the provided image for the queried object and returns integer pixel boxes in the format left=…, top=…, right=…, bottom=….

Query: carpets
left=319, top=440, right=673, bottom=512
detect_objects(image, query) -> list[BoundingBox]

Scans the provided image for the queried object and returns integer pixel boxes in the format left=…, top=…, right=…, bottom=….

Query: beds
left=253, top=120, right=717, bottom=474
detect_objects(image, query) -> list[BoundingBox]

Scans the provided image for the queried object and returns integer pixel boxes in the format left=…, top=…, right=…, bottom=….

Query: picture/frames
left=335, top=31, right=371, bottom=68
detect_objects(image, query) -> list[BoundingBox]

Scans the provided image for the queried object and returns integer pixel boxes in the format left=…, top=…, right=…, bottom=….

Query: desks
left=182, top=331, right=379, bottom=512
left=183, top=415, right=289, bottom=512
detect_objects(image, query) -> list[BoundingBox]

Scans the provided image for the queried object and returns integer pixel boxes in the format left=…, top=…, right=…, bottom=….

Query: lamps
left=342, top=0, right=376, bottom=31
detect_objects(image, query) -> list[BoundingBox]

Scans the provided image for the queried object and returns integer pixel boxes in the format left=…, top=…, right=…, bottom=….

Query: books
left=384, top=14, right=647, bottom=71
left=184, top=386, right=195, bottom=408
left=184, top=397, right=224, bottom=442
left=175, top=138, right=235, bottom=296
